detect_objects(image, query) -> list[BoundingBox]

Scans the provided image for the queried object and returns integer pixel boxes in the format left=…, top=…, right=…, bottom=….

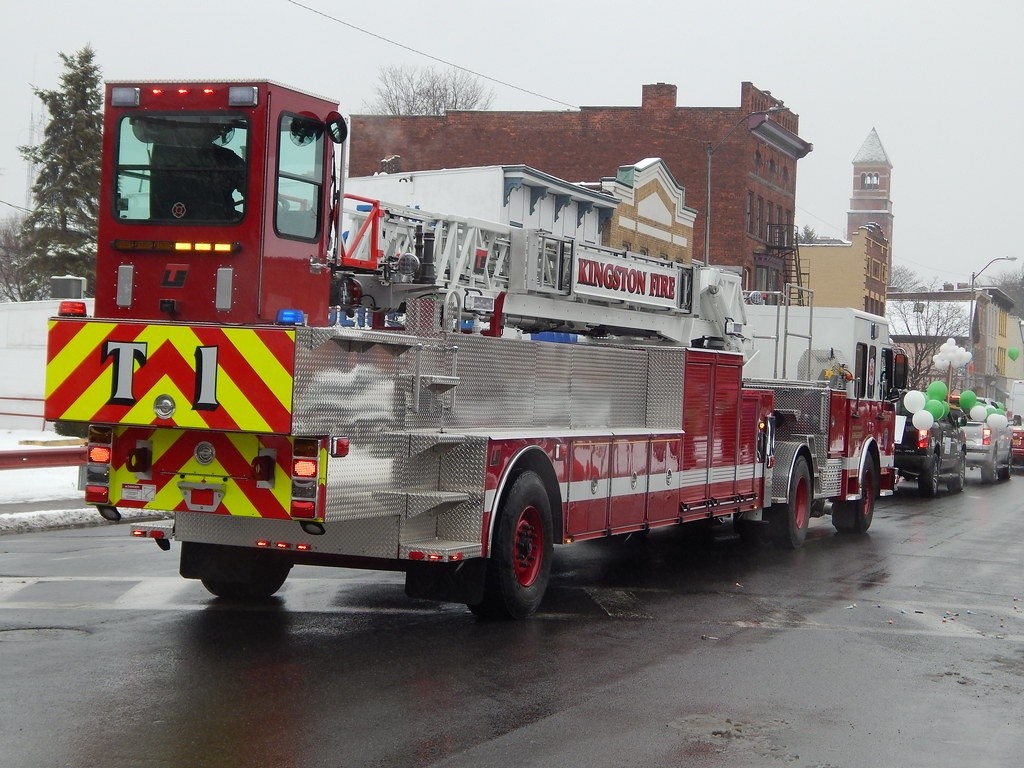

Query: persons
left=150, top=125, right=290, bottom=222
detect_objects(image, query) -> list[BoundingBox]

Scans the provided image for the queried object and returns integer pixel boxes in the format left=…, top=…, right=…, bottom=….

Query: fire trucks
left=40, top=76, right=909, bottom=625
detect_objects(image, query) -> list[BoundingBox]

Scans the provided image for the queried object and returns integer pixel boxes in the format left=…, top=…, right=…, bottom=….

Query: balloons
left=1008, top=347, right=1019, bottom=361
left=969, top=406, right=987, bottom=422
left=927, top=381, right=947, bottom=402
left=1000, top=415, right=1008, bottom=430
left=921, top=392, right=928, bottom=410
left=933, top=338, right=972, bottom=370
left=925, top=400, right=944, bottom=420
left=959, top=390, right=976, bottom=411
left=912, top=410, right=933, bottom=431
left=903, top=390, right=925, bottom=414
left=941, top=401, right=950, bottom=418
left=986, top=413, right=1001, bottom=429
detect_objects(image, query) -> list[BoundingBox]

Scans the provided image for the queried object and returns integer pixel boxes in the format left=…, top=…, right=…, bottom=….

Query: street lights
left=963, top=256, right=1018, bottom=394
left=704, top=105, right=789, bottom=266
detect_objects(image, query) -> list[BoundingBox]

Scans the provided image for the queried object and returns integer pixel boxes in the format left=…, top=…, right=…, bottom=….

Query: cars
left=959, top=396, right=1022, bottom=485
left=1008, top=418, right=1024, bottom=466
left=892, top=388, right=967, bottom=499
